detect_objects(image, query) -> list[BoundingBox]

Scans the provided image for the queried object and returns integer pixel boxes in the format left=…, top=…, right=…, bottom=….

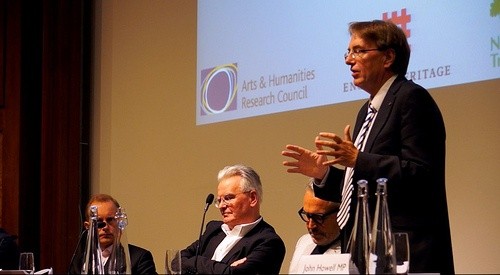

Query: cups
left=165, top=249, right=181, bottom=275
left=394, top=233, right=410, bottom=275
left=19, top=253, right=34, bottom=275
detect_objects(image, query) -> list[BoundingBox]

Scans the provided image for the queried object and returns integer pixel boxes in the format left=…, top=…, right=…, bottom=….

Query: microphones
left=193, top=193, right=214, bottom=275
left=66, top=222, right=106, bottom=275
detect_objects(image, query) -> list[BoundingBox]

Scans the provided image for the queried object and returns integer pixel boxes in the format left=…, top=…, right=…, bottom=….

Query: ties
left=336, top=103, right=379, bottom=231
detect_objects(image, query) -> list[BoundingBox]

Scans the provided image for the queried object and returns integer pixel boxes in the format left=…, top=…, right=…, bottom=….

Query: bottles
left=81, top=205, right=105, bottom=275
left=346, top=177, right=396, bottom=275
left=108, top=207, right=132, bottom=275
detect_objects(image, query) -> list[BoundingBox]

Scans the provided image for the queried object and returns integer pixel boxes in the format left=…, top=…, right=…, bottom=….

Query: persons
left=71, top=194, right=158, bottom=275
left=171, top=166, right=286, bottom=275
left=289, top=178, right=341, bottom=273
left=282, top=19, right=455, bottom=274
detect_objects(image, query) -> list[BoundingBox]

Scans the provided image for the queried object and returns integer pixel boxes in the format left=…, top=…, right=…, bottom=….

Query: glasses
left=298, top=207, right=339, bottom=225
left=344, top=48, right=379, bottom=58
left=213, top=191, right=249, bottom=209
